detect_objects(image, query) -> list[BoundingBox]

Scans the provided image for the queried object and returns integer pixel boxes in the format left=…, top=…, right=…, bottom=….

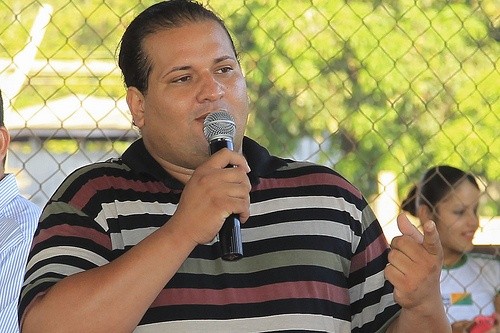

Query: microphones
left=201, top=108, right=244, bottom=263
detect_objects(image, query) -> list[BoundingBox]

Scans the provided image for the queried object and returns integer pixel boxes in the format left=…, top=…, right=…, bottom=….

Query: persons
left=401, top=165, right=500, bottom=332
left=0, top=88, right=44, bottom=333
left=17, top=0, right=454, bottom=332
